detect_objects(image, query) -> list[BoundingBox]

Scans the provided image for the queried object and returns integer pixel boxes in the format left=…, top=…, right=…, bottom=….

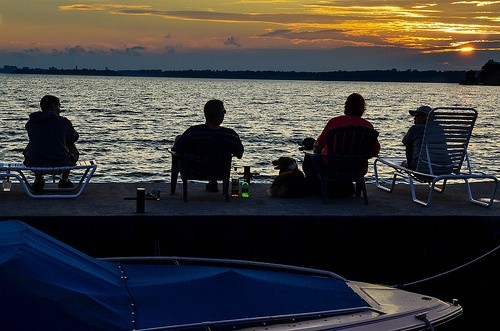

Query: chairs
left=317, top=124, right=379, bottom=205
left=374, top=106, right=498, bottom=206
left=171, top=133, right=238, bottom=202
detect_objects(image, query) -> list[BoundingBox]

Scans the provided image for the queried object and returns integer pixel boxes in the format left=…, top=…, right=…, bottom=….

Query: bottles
left=231, top=167, right=240, bottom=199
left=242, top=177, right=249, bottom=200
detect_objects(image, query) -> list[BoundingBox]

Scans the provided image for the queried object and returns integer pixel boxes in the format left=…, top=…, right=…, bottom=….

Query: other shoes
left=32, top=180, right=45, bottom=189
left=58, top=179, right=74, bottom=188
left=205, top=182, right=218, bottom=192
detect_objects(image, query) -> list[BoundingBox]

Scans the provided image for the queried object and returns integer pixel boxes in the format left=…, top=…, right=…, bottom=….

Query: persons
left=180, top=99, right=244, bottom=193
left=400, top=106, right=453, bottom=183
left=313, top=94, right=380, bottom=183
left=23, top=94, right=79, bottom=193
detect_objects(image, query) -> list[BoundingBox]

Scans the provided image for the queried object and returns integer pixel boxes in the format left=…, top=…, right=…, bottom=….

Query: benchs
left=0, top=159, right=97, bottom=199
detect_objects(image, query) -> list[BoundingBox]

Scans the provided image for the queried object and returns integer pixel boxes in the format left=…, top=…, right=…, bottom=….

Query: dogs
left=268, top=156, right=321, bottom=197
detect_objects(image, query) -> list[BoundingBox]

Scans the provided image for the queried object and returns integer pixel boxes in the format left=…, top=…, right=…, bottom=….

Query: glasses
left=218, top=110, right=226, bottom=114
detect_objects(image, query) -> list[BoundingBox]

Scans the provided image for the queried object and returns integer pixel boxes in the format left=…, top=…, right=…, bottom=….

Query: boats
left=1, top=218, right=500, bottom=331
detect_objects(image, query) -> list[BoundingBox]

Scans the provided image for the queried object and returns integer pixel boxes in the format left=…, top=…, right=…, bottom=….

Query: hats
left=409, top=106, right=434, bottom=119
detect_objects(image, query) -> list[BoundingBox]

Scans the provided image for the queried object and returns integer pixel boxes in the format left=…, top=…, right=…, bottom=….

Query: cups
left=153, top=186, right=160, bottom=200
left=3, top=181, right=11, bottom=191
